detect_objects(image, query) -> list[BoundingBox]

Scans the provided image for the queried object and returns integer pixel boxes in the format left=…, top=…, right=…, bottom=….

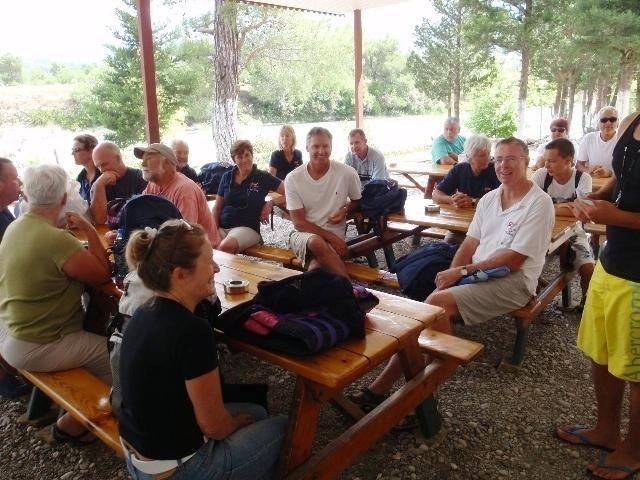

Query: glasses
left=551, top=128, right=566, bottom=132
left=600, top=117, right=617, bottom=123
left=170, top=218, right=192, bottom=262
left=72, top=147, right=85, bottom=152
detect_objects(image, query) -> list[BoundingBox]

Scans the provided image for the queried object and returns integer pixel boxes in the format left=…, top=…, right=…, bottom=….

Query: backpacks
left=106, top=193, right=183, bottom=278
left=347, top=178, right=407, bottom=239
left=390, top=241, right=457, bottom=300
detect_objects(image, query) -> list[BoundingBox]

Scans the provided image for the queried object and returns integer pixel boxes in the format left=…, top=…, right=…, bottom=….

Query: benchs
left=24, top=366, right=125, bottom=459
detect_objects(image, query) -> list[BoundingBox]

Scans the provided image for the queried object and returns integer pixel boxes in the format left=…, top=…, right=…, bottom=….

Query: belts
left=119, top=435, right=209, bottom=475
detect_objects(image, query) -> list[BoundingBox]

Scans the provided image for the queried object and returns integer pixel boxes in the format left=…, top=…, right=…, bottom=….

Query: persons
left=119, top=217, right=289, bottom=480
left=532, top=107, right=619, bottom=300
left=554, top=112, right=640, bottom=480
left=1, top=164, right=116, bottom=444
left=0, top=124, right=390, bottom=277
left=432, top=117, right=503, bottom=247
left=342, top=137, right=556, bottom=428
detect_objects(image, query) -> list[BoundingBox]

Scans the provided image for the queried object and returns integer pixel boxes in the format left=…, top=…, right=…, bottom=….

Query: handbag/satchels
left=104, top=312, right=132, bottom=418
left=197, top=162, right=233, bottom=194
left=83, top=279, right=118, bottom=337
left=107, top=197, right=128, bottom=230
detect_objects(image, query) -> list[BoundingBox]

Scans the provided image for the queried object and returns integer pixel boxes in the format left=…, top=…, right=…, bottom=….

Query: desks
left=68, top=223, right=445, bottom=479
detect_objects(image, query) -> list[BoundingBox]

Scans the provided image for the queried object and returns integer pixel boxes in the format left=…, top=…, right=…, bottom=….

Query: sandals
left=392, top=415, right=419, bottom=431
left=350, top=386, right=384, bottom=409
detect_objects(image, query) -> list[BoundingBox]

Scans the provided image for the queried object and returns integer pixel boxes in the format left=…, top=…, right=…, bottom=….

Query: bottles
left=115, top=230, right=127, bottom=278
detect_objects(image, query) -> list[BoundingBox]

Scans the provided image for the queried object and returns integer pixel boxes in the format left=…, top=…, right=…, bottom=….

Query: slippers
left=51, top=408, right=99, bottom=447
left=555, top=424, right=615, bottom=451
left=587, top=454, right=638, bottom=480
left=0, top=372, right=30, bottom=397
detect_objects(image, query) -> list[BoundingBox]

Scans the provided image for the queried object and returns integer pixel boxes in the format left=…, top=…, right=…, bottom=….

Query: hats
left=134, top=143, right=177, bottom=166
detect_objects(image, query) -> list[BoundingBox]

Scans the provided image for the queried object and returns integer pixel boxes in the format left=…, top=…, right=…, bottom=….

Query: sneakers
left=364, top=250, right=378, bottom=268
left=382, top=244, right=395, bottom=268
left=579, top=293, right=586, bottom=313
